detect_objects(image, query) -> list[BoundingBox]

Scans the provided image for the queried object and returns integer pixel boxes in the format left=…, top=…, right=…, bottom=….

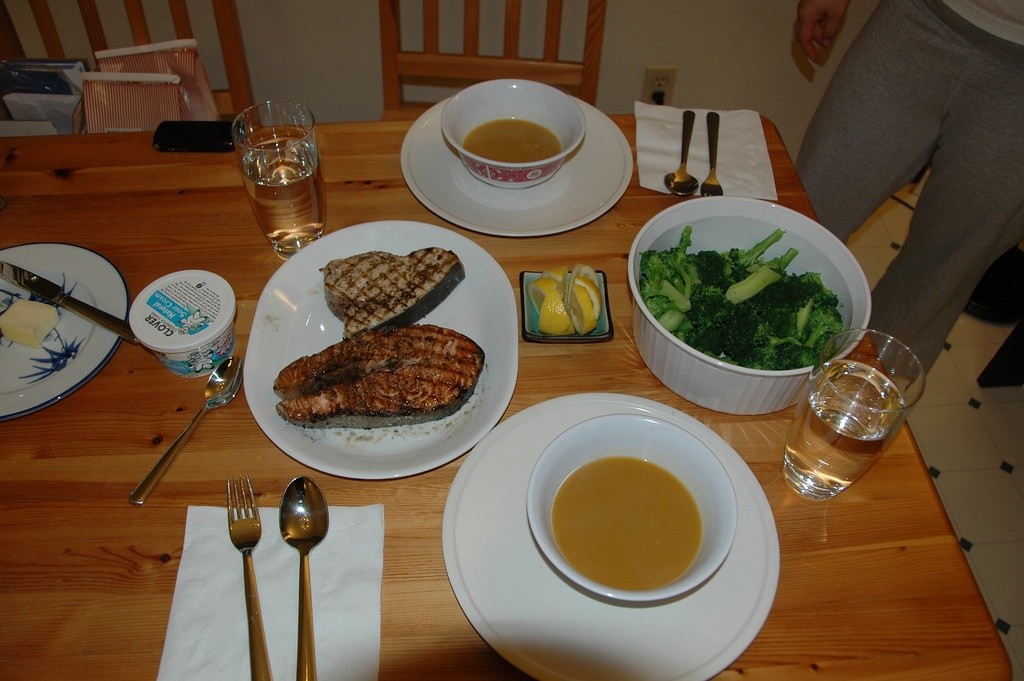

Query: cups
left=231, top=99, right=325, bottom=254
left=784, top=329, right=924, bottom=503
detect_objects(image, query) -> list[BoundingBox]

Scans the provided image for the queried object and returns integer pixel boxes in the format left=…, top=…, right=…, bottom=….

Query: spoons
left=279, top=476, right=330, bottom=681
left=663, top=110, right=699, bottom=197
left=128, top=356, right=244, bottom=506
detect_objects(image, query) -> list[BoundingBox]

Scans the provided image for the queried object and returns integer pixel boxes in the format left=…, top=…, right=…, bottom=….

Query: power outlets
left=640, top=67, right=678, bottom=107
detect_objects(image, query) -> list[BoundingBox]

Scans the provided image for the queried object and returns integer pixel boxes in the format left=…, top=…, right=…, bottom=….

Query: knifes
left=0, top=261, right=141, bottom=345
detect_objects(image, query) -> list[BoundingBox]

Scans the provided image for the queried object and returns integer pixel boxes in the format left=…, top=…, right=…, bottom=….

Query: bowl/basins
left=441, top=78, right=587, bottom=189
left=627, top=196, right=872, bottom=416
left=527, top=415, right=736, bottom=602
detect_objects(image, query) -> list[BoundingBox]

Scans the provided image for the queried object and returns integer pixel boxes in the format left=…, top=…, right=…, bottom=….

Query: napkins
left=634, top=100, right=780, bottom=201
left=157, top=503, right=384, bottom=680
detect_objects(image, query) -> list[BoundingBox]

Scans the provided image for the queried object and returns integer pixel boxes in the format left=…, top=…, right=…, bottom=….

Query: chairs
left=0, top=1, right=256, bottom=125
left=379, top=0, right=605, bottom=119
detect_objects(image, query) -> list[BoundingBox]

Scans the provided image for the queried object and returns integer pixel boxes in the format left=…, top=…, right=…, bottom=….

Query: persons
left=792, top=0, right=1023, bottom=379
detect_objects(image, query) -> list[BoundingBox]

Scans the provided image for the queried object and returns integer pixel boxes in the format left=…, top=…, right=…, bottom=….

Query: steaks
left=273, top=247, right=485, bottom=429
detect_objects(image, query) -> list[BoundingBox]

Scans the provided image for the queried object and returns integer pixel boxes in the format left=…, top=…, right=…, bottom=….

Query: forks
left=701, top=111, right=723, bottom=198
left=226, top=473, right=273, bottom=681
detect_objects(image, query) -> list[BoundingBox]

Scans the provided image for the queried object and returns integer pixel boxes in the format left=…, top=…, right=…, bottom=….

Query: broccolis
left=639, top=225, right=843, bottom=370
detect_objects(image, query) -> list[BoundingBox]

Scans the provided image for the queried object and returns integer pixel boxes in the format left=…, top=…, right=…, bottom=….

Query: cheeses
left=0, top=299, right=59, bottom=349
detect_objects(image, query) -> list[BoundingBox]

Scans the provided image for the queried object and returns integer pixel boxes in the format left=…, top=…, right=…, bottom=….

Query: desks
left=0, top=108, right=1013, bottom=680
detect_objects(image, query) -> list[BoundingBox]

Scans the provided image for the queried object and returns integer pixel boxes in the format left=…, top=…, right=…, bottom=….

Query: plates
left=440, top=392, right=780, bottom=681
left=0, top=245, right=128, bottom=420
left=244, top=222, right=521, bottom=482
left=520, top=269, right=615, bottom=345
left=400, top=97, right=632, bottom=237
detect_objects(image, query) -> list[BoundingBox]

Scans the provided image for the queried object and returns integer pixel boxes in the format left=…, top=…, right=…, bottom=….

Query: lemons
left=527, top=265, right=601, bottom=336
